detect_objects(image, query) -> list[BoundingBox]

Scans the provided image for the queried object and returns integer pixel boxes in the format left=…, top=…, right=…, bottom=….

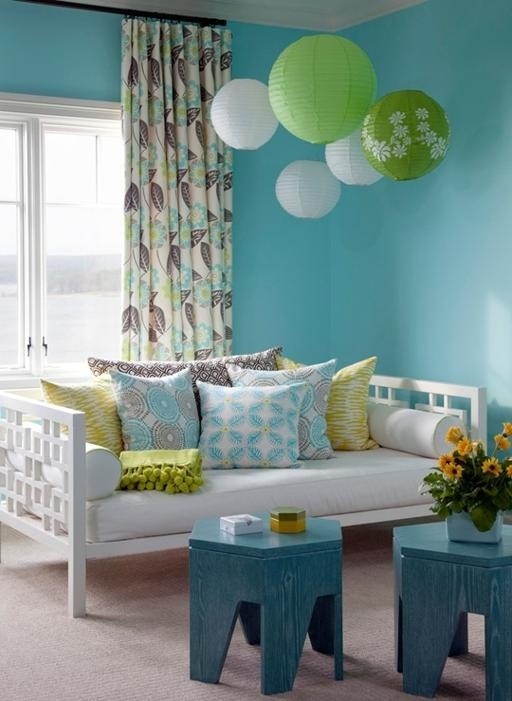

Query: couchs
left=0, top=373, right=492, bottom=621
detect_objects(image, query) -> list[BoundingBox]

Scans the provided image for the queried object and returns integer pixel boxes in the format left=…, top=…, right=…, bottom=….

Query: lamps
left=210, top=32, right=450, bottom=219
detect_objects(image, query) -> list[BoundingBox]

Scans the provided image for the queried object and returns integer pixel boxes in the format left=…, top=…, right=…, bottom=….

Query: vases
left=444, top=498, right=501, bottom=544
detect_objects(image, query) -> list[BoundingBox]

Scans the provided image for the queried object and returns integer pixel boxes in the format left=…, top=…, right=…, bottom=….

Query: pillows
left=190, top=377, right=306, bottom=472
left=84, top=344, right=286, bottom=434
left=223, top=358, right=341, bottom=460
left=276, top=355, right=380, bottom=452
left=105, top=367, right=205, bottom=455
left=42, top=371, right=128, bottom=455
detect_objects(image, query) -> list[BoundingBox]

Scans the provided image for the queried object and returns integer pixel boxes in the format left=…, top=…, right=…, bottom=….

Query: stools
left=186, top=511, right=345, bottom=695
left=390, top=519, right=512, bottom=700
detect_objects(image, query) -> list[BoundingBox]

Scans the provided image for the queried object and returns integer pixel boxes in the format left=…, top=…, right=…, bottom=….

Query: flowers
left=417, top=420, right=511, bottom=533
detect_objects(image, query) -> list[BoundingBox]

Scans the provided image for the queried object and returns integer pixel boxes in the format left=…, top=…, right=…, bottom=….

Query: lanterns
left=275, top=159, right=342, bottom=220
left=268, top=34, right=377, bottom=145
left=360, top=89, right=451, bottom=182
left=325, top=128, right=385, bottom=186
left=211, top=78, right=279, bottom=151
